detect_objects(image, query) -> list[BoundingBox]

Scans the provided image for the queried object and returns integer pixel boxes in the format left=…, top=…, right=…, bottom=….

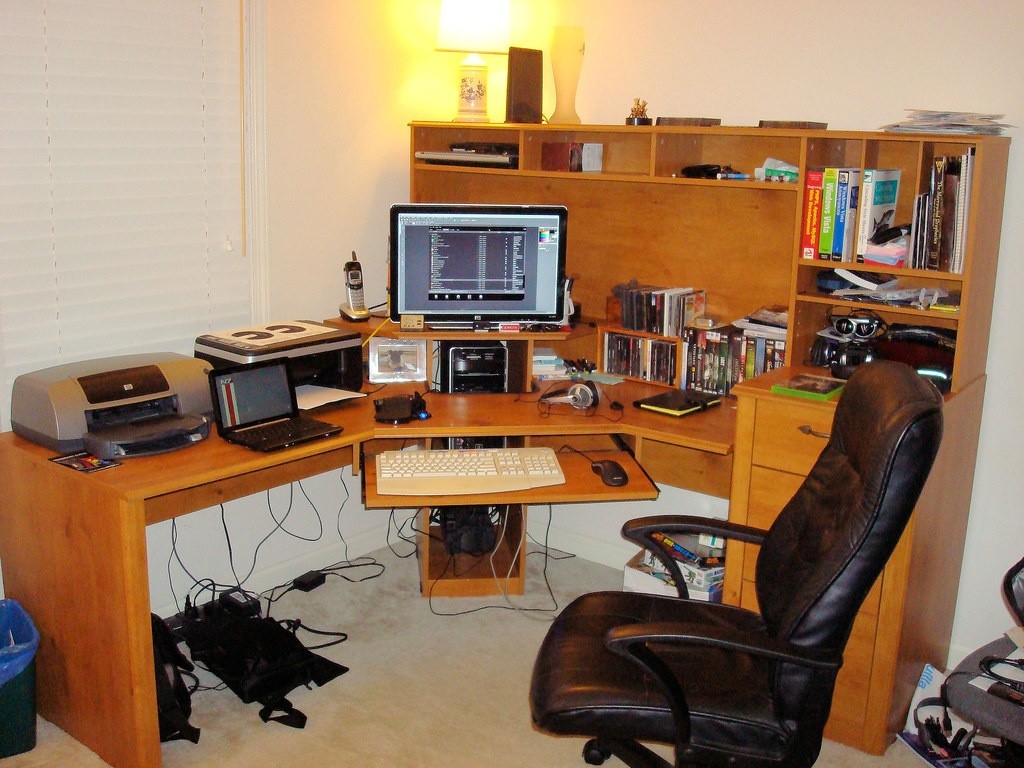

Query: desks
left=0, top=373, right=994, bottom=768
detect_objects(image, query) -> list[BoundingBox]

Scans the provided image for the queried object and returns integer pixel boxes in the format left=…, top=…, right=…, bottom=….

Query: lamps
left=434, top=2, right=513, bottom=122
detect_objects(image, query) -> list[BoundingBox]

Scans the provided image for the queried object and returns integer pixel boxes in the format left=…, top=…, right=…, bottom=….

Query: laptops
left=208, top=356, right=344, bottom=453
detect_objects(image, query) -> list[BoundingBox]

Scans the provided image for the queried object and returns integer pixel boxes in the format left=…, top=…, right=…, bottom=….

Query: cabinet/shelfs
left=407, top=119, right=1011, bottom=394
left=724, top=369, right=988, bottom=754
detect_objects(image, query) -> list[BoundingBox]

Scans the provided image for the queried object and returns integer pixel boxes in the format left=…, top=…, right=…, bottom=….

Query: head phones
left=540, top=379, right=602, bottom=409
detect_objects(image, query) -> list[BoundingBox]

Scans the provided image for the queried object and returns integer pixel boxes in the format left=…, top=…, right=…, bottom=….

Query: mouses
left=591, top=460, right=628, bottom=486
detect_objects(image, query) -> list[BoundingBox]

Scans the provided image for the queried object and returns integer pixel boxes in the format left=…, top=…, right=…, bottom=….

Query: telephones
left=339, top=249, right=371, bottom=323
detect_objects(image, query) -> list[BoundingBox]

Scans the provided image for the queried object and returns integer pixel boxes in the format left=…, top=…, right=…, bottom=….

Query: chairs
left=528, top=361, right=945, bottom=767
left=943, top=558, right=1024, bottom=768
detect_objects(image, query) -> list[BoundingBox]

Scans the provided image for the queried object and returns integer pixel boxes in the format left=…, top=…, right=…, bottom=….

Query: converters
left=219, top=588, right=262, bottom=618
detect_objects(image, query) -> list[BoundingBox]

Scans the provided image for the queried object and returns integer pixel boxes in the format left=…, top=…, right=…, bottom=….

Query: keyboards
left=375, top=446, right=566, bottom=494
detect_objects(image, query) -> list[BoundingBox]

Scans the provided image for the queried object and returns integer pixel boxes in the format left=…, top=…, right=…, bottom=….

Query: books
left=834, top=267, right=897, bottom=291
left=910, top=146, right=975, bottom=274
left=533, top=347, right=571, bottom=381
left=603, top=284, right=788, bottom=399
left=798, top=166, right=902, bottom=264
left=632, top=390, right=721, bottom=418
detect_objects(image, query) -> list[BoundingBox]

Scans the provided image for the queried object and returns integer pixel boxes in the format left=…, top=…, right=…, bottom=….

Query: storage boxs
left=621, top=516, right=731, bottom=602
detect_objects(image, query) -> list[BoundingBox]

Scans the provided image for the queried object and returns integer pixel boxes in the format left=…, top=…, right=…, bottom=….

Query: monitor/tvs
left=388, top=204, right=568, bottom=333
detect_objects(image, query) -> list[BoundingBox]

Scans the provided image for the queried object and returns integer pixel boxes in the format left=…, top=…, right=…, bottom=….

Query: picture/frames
left=369, top=339, right=427, bottom=383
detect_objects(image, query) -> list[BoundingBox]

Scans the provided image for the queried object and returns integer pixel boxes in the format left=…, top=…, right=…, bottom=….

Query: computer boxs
left=440, top=339, right=509, bottom=451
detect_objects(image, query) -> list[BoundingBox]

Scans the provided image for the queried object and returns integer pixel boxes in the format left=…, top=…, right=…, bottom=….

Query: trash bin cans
left=1, top=595, right=40, bottom=761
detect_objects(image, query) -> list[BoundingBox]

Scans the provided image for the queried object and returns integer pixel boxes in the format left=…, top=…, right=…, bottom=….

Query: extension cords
left=165, top=599, right=228, bottom=633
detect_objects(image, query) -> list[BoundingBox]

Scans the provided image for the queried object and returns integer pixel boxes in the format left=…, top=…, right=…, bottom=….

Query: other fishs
left=867, top=210, right=894, bottom=242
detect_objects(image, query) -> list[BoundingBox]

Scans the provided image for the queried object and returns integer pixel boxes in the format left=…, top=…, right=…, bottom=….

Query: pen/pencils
left=716, top=172, right=752, bottom=181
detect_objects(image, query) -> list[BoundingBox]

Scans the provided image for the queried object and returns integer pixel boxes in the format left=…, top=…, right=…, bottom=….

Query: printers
left=194, top=321, right=368, bottom=421
left=10, top=352, right=215, bottom=460
left=293, top=570, right=326, bottom=592
